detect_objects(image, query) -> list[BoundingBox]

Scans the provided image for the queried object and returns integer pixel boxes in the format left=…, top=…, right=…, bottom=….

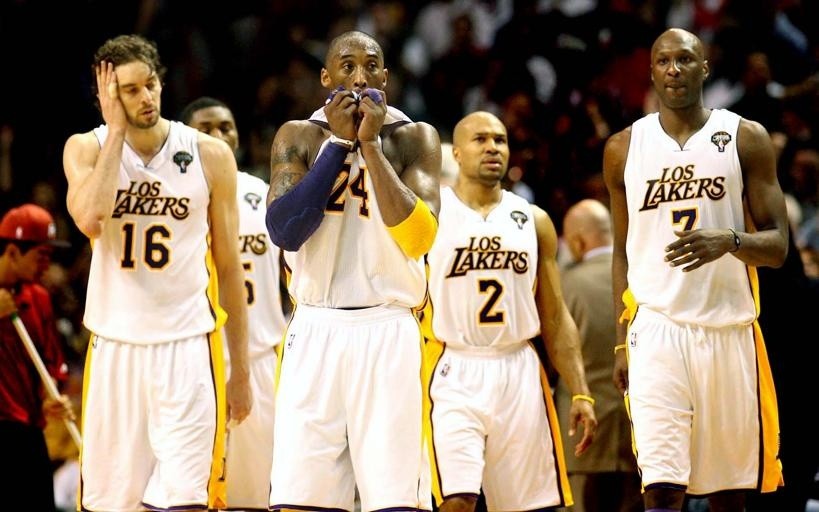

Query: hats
left=1, top=204, right=70, bottom=247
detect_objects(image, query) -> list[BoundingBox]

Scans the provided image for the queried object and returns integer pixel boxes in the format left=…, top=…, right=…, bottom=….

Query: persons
left=0, top=0, right=819, bottom=512
